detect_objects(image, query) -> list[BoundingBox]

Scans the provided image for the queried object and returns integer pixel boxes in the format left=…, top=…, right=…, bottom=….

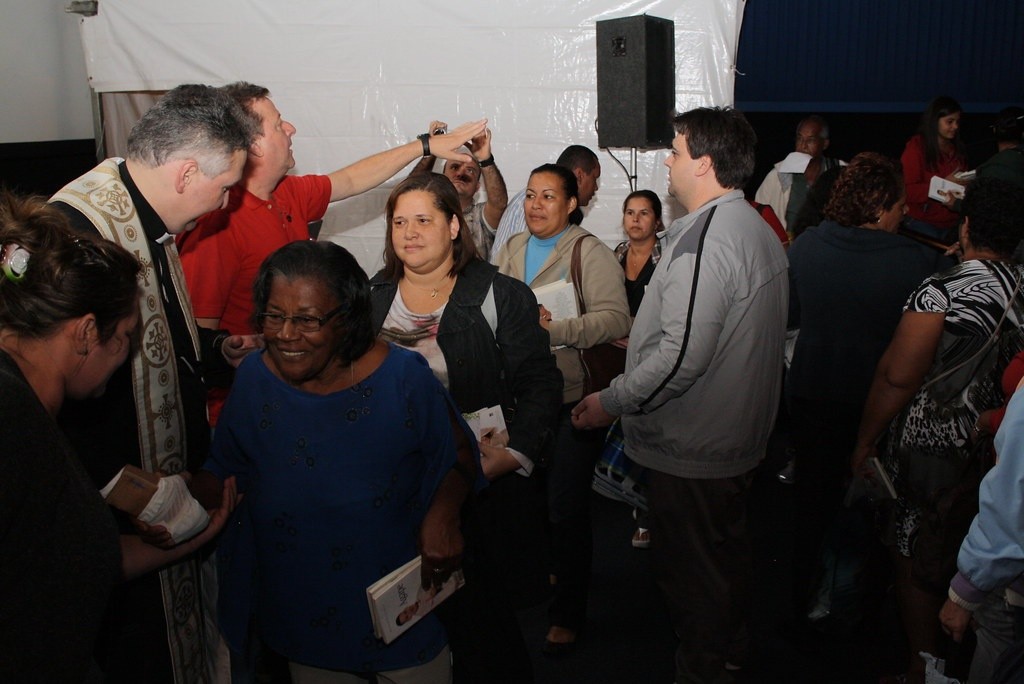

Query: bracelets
left=478, top=154, right=494, bottom=167
left=948, top=586, right=980, bottom=612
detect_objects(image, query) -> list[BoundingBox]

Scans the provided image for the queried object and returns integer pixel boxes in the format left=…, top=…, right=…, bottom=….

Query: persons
left=591, top=189, right=662, bottom=391
left=42, top=83, right=265, bottom=684
left=944, top=107, right=1024, bottom=262
left=793, top=157, right=949, bottom=641
left=853, top=163, right=1024, bottom=684
left=939, top=383, right=1024, bottom=684
left=493, top=163, right=632, bottom=655
left=900, top=96, right=969, bottom=236
left=0, top=185, right=242, bottom=684
left=571, top=105, right=790, bottom=684
left=754, top=115, right=850, bottom=233
left=396, top=571, right=465, bottom=626
left=938, top=189, right=962, bottom=200
left=488, top=145, right=601, bottom=267
left=205, top=239, right=484, bottom=684
left=410, top=120, right=508, bottom=261
left=368, top=170, right=565, bottom=684
left=175, top=81, right=488, bottom=431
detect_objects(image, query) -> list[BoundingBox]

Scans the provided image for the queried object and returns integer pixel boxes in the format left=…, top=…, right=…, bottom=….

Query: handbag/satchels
left=569, top=231, right=629, bottom=434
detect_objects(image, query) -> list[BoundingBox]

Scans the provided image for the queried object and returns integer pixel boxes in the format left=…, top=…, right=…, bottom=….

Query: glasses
left=254, top=300, right=353, bottom=333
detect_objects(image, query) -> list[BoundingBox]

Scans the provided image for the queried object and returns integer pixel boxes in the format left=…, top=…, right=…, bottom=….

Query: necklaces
left=431, top=287, right=438, bottom=298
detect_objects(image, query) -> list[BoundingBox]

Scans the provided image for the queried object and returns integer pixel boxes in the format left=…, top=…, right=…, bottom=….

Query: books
left=955, top=170, right=976, bottom=180
left=366, top=554, right=465, bottom=645
left=475, top=404, right=509, bottom=457
left=532, top=279, right=578, bottom=351
left=928, top=176, right=965, bottom=203
left=865, top=456, right=897, bottom=499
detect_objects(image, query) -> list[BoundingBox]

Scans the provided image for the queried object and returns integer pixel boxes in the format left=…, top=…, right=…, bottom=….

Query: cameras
left=433, top=129, right=446, bottom=135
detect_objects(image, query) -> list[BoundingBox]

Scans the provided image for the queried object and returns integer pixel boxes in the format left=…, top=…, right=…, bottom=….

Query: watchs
left=417, top=133, right=430, bottom=156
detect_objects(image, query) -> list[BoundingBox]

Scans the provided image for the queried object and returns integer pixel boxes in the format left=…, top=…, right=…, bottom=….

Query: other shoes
left=632, top=528, right=651, bottom=548
left=879, top=667, right=926, bottom=684
left=541, top=624, right=578, bottom=657
left=724, top=654, right=745, bottom=671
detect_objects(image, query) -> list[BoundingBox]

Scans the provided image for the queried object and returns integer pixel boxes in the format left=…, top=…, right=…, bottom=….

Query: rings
left=432, top=568, right=442, bottom=573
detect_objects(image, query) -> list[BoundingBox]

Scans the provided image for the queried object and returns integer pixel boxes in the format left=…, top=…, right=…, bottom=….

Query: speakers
left=595, top=13, right=676, bottom=154
